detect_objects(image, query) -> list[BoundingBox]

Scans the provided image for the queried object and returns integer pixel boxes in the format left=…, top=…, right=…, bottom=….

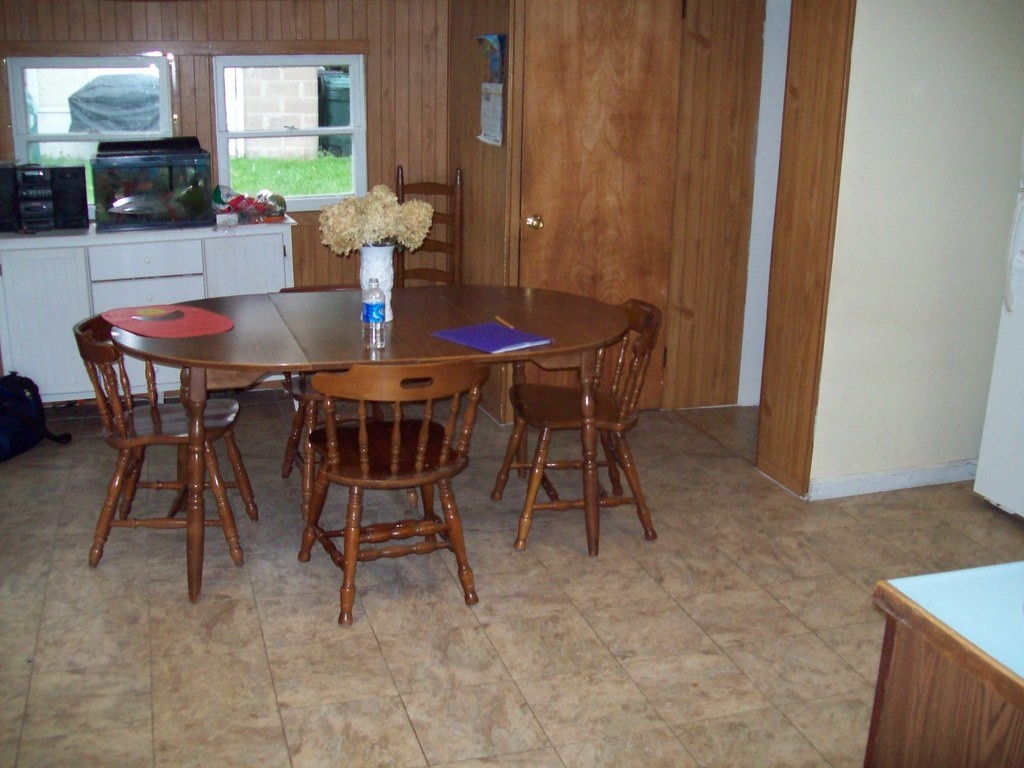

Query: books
left=429, top=322, right=552, bottom=354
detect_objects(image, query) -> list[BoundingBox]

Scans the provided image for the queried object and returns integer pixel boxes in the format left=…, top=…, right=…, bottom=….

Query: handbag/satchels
left=0, top=373, right=72, bottom=462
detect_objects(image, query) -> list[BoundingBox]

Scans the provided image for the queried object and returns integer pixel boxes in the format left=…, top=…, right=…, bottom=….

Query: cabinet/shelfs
left=0, top=226, right=299, bottom=406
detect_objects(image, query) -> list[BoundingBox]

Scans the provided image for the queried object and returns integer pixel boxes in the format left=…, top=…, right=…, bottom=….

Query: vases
left=358, top=243, right=395, bottom=322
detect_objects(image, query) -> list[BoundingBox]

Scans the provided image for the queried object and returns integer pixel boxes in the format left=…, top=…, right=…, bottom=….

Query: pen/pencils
left=495, top=316, right=514, bottom=330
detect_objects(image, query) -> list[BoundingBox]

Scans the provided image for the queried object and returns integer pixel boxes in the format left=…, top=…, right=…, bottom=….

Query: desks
left=863, top=558, right=1024, bottom=768
left=97, top=284, right=630, bottom=510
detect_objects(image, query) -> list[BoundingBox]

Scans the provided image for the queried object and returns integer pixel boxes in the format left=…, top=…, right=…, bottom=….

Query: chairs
left=298, top=361, right=490, bottom=627
left=492, top=297, right=662, bottom=551
left=395, top=164, right=464, bottom=288
left=278, top=286, right=384, bottom=520
left=74, top=316, right=260, bottom=566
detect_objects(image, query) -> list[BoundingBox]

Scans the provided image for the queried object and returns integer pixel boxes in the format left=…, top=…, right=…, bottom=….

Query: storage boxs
left=89, top=149, right=215, bottom=233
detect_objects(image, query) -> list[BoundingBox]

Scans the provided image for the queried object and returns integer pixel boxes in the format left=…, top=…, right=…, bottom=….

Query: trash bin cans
left=318, top=69, right=352, bottom=158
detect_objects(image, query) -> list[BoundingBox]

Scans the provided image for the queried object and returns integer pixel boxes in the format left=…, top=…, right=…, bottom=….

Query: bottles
left=360, top=279, right=386, bottom=349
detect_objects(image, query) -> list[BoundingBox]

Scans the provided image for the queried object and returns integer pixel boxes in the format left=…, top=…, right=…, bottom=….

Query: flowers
left=317, top=184, right=433, bottom=255
